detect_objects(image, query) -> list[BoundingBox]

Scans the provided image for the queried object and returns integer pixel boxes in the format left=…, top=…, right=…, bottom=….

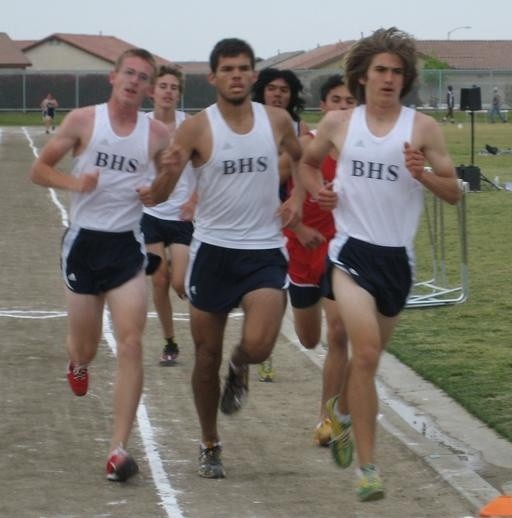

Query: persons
left=442, top=85, right=458, bottom=124
left=274, top=75, right=360, bottom=446
left=143, top=60, right=198, bottom=368
left=149, top=37, right=304, bottom=477
left=298, top=26, right=462, bottom=501
left=250, top=67, right=306, bottom=381
left=491, top=85, right=509, bottom=124
left=42, top=93, right=58, bottom=133
left=32, top=49, right=171, bottom=481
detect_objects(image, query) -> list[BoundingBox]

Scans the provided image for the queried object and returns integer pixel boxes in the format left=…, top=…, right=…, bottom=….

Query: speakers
left=460, top=87, right=481, bottom=111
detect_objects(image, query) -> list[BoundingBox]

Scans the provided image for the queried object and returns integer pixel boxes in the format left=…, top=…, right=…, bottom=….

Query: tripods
left=468, top=111, right=503, bottom=190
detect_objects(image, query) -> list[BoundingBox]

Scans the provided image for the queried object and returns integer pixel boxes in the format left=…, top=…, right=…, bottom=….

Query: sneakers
left=219, top=344, right=248, bottom=414
left=160, top=342, right=181, bottom=366
left=45, top=126, right=55, bottom=135
left=107, top=445, right=138, bottom=481
left=317, top=394, right=386, bottom=501
left=199, top=442, right=225, bottom=478
left=259, top=356, right=273, bottom=382
left=68, top=361, right=88, bottom=397
